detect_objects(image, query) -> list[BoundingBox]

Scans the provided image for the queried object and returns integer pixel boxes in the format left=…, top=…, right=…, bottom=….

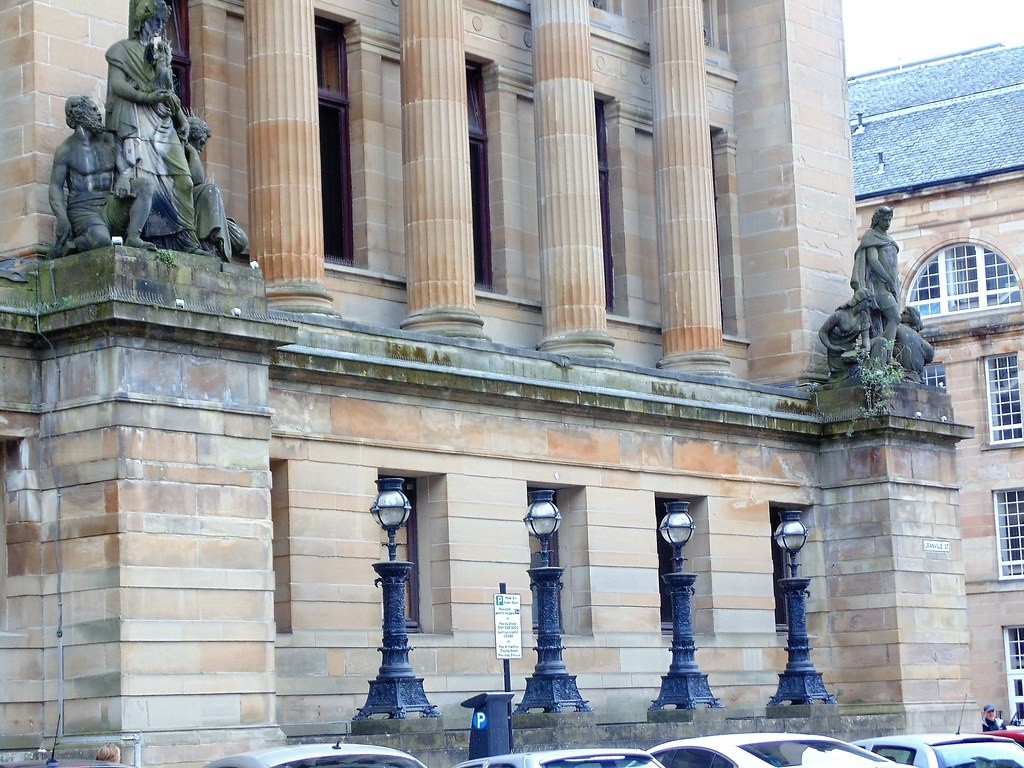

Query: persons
left=817, top=205, right=934, bottom=384
left=104, top=0, right=209, bottom=256
left=46, top=95, right=157, bottom=262
left=184, top=116, right=232, bottom=261
left=983, top=704, right=1007, bottom=732
left=97, top=744, right=121, bottom=764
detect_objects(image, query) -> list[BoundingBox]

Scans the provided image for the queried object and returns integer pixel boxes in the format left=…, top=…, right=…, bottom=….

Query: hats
left=984, top=704, right=995, bottom=712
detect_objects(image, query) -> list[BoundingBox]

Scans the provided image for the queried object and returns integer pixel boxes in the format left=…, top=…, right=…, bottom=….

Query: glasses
left=988, top=710, right=995, bottom=713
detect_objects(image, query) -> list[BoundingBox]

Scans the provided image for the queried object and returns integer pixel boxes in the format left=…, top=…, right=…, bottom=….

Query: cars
left=841, top=733, right=1024, bottom=768
left=976, top=729, right=1024, bottom=749
left=201, top=740, right=427, bottom=768
left=622, top=729, right=918, bottom=768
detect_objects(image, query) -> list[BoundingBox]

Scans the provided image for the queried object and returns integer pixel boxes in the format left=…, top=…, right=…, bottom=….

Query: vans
left=453, top=749, right=665, bottom=768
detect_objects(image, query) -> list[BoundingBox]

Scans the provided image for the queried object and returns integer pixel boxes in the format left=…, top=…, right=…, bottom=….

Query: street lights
left=351, top=477, right=445, bottom=718
left=765, top=509, right=838, bottom=705
left=513, top=488, right=594, bottom=714
left=648, top=499, right=724, bottom=707
